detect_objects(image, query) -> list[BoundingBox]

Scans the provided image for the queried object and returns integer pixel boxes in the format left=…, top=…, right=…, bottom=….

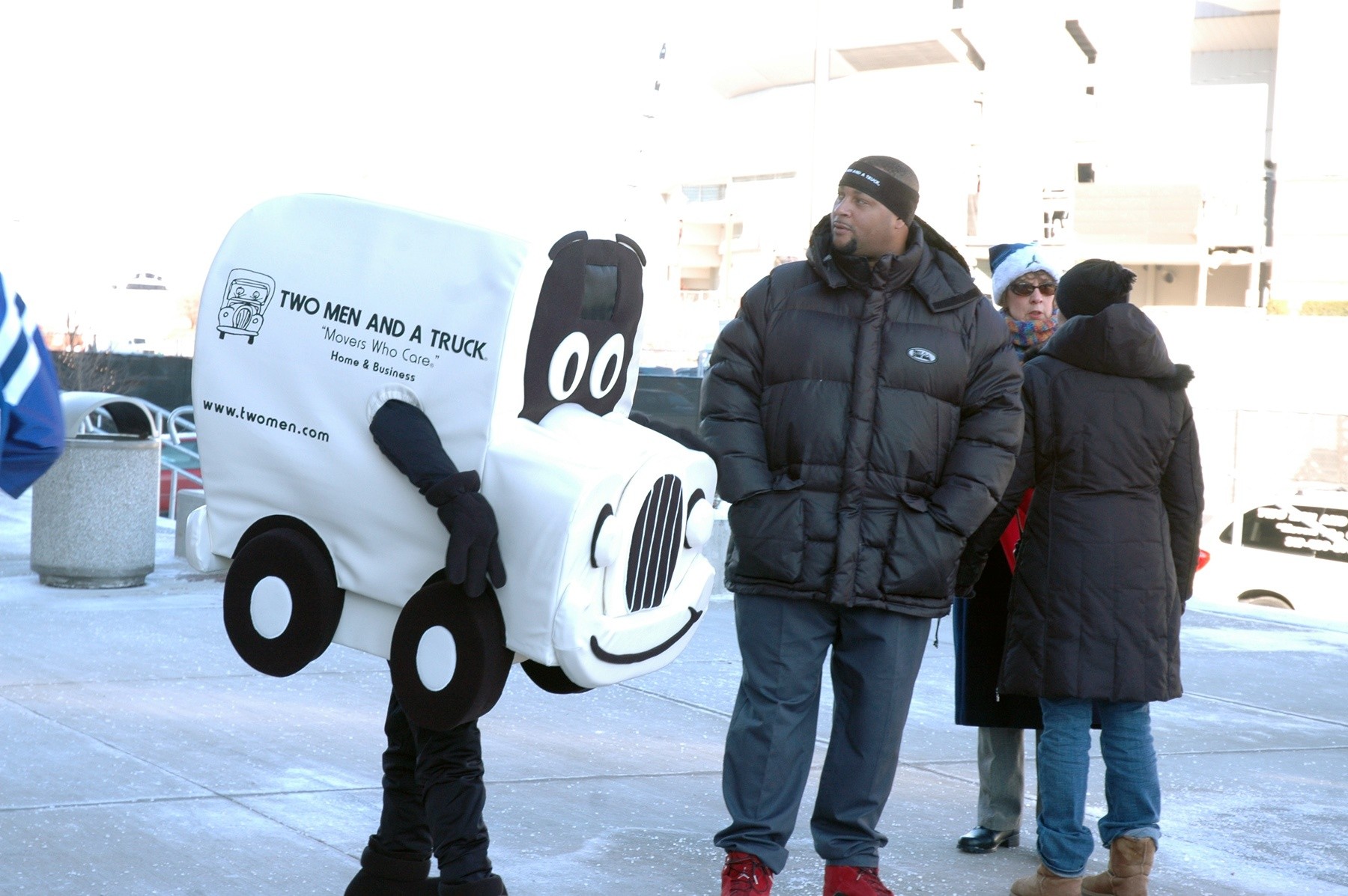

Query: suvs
left=1187, top=491, right=1348, bottom=621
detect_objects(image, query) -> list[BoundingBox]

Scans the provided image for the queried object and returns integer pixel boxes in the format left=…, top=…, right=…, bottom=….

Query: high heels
left=957, top=825, right=1019, bottom=853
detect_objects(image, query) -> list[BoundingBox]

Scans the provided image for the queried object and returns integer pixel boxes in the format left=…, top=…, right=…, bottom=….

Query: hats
left=1055, top=258, right=1137, bottom=320
left=988, top=243, right=1061, bottom=307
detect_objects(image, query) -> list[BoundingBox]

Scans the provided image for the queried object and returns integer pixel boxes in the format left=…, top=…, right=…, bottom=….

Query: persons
left=697, top=155, right=1025, bottom=895
left=1012, top=258, right=1204, bottom=895
left=952, top=243, right=1060, bottom=853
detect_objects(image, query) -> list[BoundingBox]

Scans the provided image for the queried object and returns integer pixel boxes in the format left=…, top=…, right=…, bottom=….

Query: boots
left=1082, top=837, right=1156, bottom=896
left=1010, top=863, right=1083, bottom=896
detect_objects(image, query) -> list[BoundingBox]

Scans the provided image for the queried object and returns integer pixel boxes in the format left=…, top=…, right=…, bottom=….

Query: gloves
left=952, top=536, right=988, bottom=597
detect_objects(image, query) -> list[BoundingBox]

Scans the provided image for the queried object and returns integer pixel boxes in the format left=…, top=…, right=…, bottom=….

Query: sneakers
left=823, top=865, right=896, bottom=896
left=720, top=850, right=774, bottom=896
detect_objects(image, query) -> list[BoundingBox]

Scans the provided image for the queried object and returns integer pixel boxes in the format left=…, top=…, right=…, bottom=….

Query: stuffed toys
left=191, top=196, right=717, bottom=895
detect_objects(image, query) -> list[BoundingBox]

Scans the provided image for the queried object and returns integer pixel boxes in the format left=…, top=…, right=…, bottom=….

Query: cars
left=159, top=433, right=204, bottom=514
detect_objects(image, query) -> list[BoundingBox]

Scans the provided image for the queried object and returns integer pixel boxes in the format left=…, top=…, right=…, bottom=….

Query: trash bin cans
left=28, top=390, right=163, bottom=592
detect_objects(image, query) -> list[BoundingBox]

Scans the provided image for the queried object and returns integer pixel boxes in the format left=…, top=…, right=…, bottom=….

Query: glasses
left=1008, top=282, right=1058, bottom=296
